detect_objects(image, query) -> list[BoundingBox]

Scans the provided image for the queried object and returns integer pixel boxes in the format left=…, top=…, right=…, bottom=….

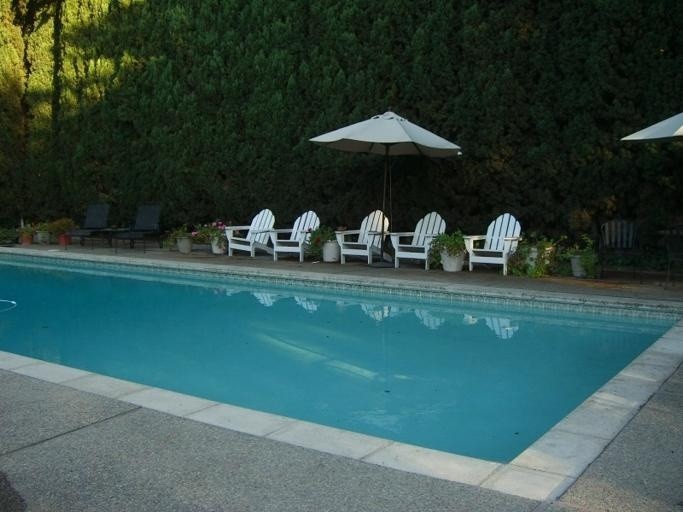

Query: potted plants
left=190, top=221, right=226, bottom=254
left=160, top=227, right=198, bottom=253
left=428, top=229, right=468, bottom=272
left=15, top=223, right=35, bottom=245
left=36, top=219, right=51, bottom=244
left=54, top=219, right=78, bottom=245
left=310, top=225, right=340, bottom=262
left=508, top=235, right=554, bottom=277
left=569, top=234, right=601, bottom=279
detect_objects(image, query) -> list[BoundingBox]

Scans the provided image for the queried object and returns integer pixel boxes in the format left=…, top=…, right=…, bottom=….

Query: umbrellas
left=307, top=110, right=462, bottom=261
left=618, top=112, right=683, bottom=143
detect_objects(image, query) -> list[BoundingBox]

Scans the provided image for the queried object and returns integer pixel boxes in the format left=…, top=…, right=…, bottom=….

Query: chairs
left=462, top=213, right=521, bottom=275
left=225, top=208, right=275, bottom=257
left=334, top=210, right=392, bottom=264
left=113, top=205, right=161, bottom=253
left=271, top=211, right=320, bottom=263
left=389, top=211, right=447, bottom=270
left=69, top=203, right=108, bottom=246
